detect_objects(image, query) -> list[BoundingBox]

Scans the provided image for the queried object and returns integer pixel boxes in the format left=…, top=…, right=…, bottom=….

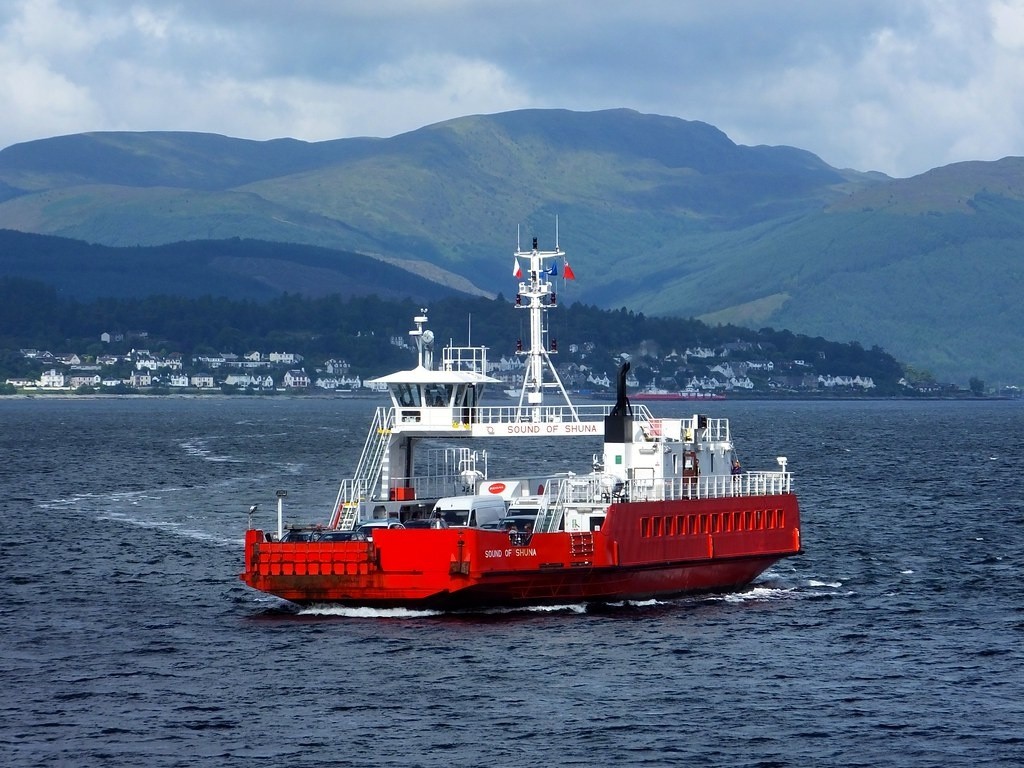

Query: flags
left=513, top=258, right=523, bottom=278
left=545, top=260, right=559, bottom=275
left=562, top=263, right=576, bottom=279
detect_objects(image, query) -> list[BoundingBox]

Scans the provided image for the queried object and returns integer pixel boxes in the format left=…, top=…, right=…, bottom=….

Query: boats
left=239, top=214, right=806, bottom=615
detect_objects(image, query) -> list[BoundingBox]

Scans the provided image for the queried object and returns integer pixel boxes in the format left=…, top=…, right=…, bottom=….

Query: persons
left=732, top=462, right=745, bottom=476
